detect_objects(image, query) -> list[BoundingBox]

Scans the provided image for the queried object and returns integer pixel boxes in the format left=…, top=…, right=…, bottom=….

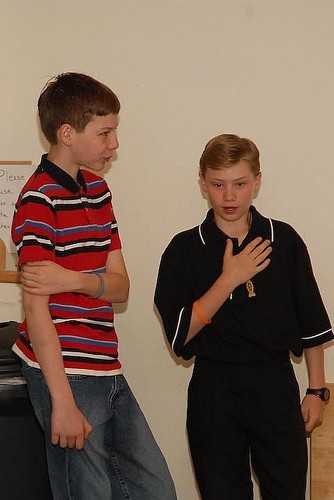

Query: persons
left=153, top=134, right=334, bottom=500
left=11, top=72, right=179, bottom=500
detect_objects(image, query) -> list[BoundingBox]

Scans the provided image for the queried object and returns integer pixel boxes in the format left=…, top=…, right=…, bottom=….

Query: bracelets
left=194, top=300, right=212, bottom=325
left=89, top=272, right=105, bottom=298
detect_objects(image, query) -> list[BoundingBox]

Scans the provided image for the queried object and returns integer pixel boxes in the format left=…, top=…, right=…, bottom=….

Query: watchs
left=307, top=387, right=331, bottom=401
